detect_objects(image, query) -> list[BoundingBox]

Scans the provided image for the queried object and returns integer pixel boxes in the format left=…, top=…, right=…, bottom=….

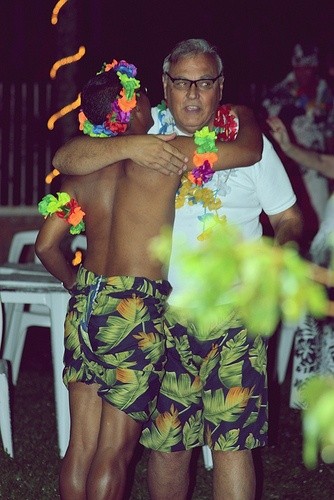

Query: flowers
left=37, top=59, right=239, bottom=236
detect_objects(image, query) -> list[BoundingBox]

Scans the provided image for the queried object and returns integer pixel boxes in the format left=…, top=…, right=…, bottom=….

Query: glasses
left=165, top=71, right=223, bottom=89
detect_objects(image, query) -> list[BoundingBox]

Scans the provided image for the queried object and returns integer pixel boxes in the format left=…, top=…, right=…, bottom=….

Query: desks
left=0, top=263, right=72, bottom=459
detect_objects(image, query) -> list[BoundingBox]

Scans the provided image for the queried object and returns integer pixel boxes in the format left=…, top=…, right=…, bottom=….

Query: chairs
left=0, top=228, right=214, bottom=473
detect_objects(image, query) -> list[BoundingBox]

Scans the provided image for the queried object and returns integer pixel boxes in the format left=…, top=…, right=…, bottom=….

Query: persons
left=265, top=33, right=334, bottom=434
left=32, top=71, right=265, bottom=500
left=53, top=38, right=308, bottom=500
